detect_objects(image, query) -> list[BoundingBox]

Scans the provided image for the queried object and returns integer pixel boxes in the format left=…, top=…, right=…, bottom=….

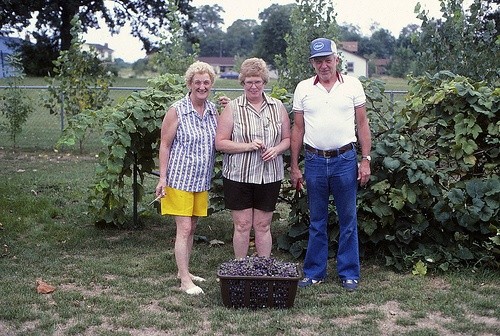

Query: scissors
left=147, top=189, right=165, bottom=208
left=296, top=179, right=303, bottom=199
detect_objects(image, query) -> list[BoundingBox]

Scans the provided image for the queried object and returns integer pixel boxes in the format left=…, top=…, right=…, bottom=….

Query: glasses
left=244, top=80, right=266, bottom=86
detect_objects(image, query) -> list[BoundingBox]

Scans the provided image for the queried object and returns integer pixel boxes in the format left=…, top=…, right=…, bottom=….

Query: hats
left=310, top=38, right=337, bottom=58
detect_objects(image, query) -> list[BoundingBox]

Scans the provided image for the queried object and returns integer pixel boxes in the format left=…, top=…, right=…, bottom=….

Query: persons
left=155, top=60, right=231, bottom=296
left=215, top=38, right=371, bottom=290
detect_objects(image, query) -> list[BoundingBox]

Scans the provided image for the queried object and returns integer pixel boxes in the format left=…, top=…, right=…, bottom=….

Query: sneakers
left=343, top=280, right=359, bottom=291
left=298, top=278, right=327, bottom=288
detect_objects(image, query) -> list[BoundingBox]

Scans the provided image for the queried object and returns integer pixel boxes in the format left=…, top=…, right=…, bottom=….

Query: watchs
left=362, top=155, right=372, bottom=161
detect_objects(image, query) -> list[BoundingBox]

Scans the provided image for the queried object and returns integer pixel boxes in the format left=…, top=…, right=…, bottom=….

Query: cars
left=221, top=70, right=240, bottom=81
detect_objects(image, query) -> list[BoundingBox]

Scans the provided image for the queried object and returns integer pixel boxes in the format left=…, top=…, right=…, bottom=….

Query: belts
left=305, top=143, right=353, bottom=159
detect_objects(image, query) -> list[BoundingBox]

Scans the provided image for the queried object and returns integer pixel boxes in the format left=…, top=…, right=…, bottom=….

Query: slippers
left=181, top=286, right=204, bottom=294
left=191, top=276, right=206, bottom=281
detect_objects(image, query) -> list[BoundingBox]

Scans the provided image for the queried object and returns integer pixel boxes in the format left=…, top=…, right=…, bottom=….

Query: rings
left=271, top=155, right=273, bottom=157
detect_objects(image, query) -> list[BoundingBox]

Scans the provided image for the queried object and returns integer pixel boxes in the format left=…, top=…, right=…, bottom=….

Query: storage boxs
left=217, top=263, right=303, bottom=311
